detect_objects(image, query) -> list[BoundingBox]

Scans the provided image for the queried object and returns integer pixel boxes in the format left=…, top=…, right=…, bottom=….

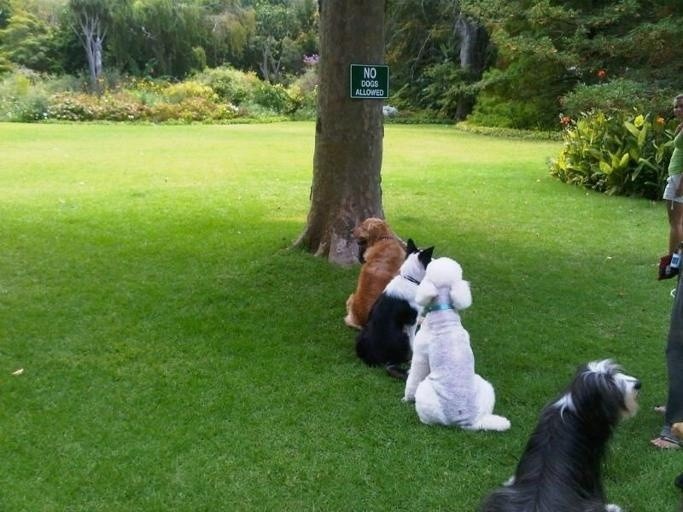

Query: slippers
left=650, top=432, right=682, bottom=452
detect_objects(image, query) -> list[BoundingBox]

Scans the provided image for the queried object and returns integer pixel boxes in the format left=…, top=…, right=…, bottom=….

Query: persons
left=646, top=242, right=683, bottom=452
left=660, top=95, right=682, bottom=257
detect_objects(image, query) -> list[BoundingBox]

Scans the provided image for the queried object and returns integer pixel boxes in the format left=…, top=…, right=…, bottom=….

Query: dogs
left=476, top=358, right=641, bottom=511
left=355, top=239, right=439, bottom=385
left=343, top=218, right=409, bottom=331
left=400, top=257, right=511, bottom=434
